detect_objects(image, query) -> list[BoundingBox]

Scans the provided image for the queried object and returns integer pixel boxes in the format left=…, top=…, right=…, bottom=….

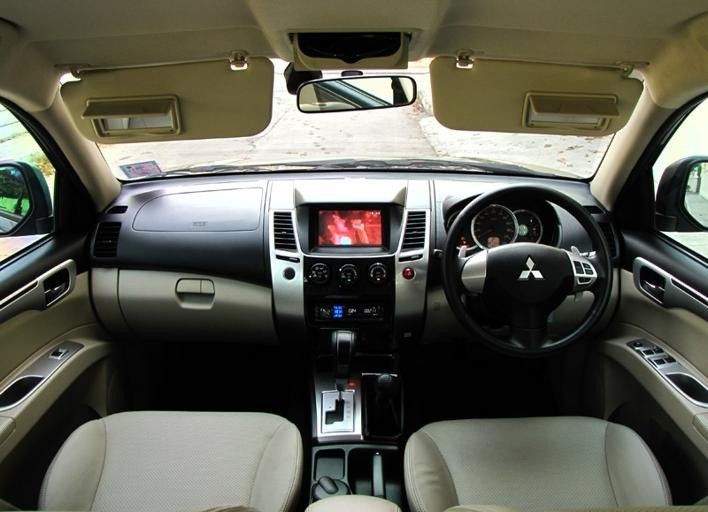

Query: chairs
left=403, top=415, right=707, bottom=511
left=37, top=410, right=303, bottom=512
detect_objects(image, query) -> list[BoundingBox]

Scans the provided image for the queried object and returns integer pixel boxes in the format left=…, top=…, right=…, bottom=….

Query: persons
left=319, top=212, right=370, bottom=245
